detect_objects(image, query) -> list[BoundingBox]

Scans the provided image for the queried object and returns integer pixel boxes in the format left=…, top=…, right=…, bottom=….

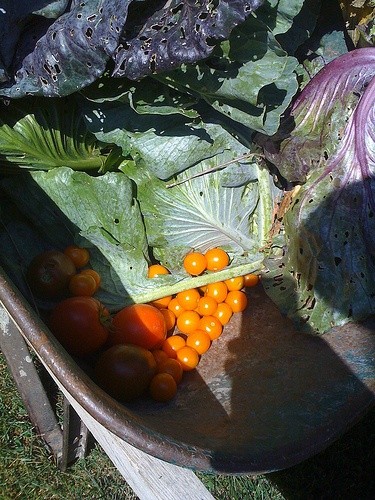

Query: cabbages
left=0, top=0, right=375, bottom=336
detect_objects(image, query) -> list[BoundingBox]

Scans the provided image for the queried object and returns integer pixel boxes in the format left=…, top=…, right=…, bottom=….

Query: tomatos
left=27, top=244, right=257, bottom=404
